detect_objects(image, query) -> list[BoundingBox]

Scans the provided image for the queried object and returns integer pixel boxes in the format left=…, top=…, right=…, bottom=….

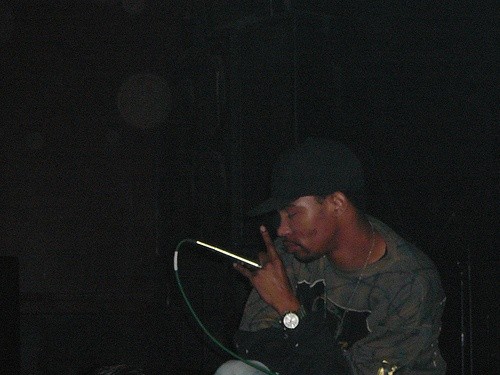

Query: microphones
left=187, top=239, right=263, bottom=271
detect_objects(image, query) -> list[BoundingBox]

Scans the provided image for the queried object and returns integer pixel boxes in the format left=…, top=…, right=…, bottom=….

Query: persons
left=213, top=136, right=448, bottom=375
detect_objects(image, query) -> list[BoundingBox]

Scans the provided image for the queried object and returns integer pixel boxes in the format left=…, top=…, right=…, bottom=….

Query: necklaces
left=322, top=215, right=376, bottom=339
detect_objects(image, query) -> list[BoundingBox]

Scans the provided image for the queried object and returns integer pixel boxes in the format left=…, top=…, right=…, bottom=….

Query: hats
left=255, top=140, right=363, bottom=216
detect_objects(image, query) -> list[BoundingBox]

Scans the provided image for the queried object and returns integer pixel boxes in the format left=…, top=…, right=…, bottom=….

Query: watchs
left=277, top=305, right=306, bottom=331
